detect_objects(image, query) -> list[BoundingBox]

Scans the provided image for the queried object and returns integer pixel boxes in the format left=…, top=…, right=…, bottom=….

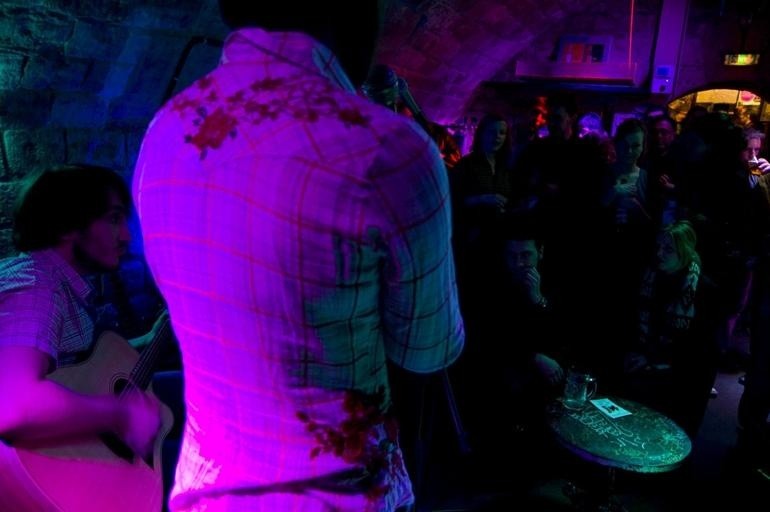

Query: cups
left=749, top=156, right=762, bottom=179
left=559, top=365, right=599, bottom=413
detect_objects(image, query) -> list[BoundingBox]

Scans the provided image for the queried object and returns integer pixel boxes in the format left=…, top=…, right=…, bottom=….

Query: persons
left=449, top=105, right=769, bottom=428
left=0, top=164, right=164, bottom=455
left=131, top=1, right=466, bottom=512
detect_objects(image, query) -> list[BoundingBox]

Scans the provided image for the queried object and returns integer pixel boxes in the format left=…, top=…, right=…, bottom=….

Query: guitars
left=0, top=299, right=175, bottom=512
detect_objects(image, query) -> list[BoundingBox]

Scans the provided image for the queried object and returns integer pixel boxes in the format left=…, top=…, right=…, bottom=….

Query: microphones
left=362, top=62, right=401, bottom=115
left=398, top=77, right=429, bottom=133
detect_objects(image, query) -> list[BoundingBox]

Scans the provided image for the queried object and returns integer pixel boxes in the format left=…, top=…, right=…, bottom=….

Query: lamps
left=720, top=0, right=761, bottom=67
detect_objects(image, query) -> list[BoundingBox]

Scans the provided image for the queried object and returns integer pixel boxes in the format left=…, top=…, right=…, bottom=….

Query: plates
left=588, top=398, right=633, bottom=420
